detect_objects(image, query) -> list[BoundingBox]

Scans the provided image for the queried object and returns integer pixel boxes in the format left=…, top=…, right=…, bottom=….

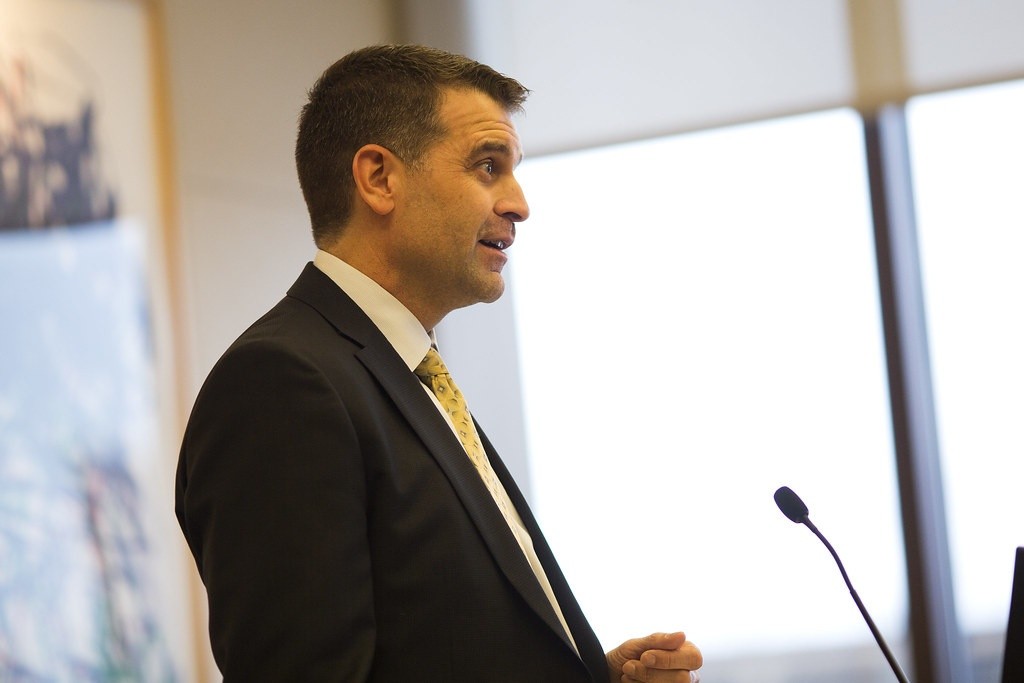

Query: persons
left=173, top=43, right=711, bottom=683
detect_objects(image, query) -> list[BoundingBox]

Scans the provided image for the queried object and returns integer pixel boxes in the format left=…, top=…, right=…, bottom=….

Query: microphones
left=774, top=485, right=911, bottom=683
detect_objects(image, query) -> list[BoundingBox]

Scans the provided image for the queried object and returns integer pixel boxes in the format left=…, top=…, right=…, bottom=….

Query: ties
left=414, top=347, right=521, bottom=535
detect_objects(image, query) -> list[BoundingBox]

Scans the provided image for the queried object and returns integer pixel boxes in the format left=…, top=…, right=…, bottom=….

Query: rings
left=689, top=670, right=698, bottom=682
